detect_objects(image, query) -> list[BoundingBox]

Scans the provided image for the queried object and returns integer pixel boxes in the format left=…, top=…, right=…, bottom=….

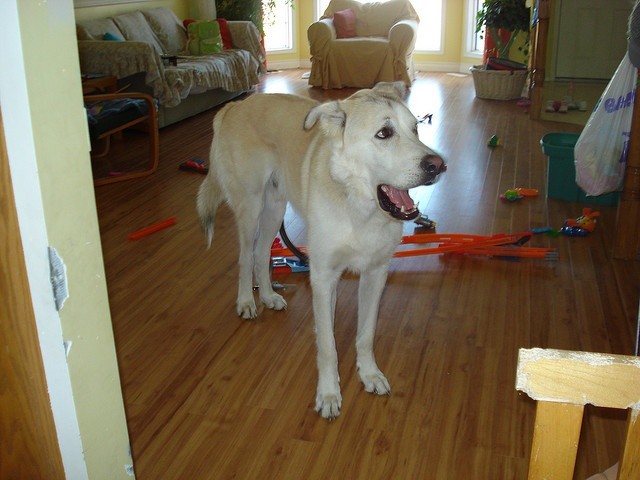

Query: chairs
left=82, top=76, right=158, bottom=188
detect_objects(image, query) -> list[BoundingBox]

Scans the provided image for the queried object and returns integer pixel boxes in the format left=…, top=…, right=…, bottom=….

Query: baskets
left=469, top=65, right=529, bottom=101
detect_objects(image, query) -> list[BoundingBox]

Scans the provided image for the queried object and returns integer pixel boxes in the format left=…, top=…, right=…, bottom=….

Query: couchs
left=307, top=0, right=420, bottom=90
left=77, top=7, right=266, bottom=130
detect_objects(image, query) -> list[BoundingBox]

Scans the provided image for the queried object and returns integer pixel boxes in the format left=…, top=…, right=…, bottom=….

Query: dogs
left=194, top=80, right=447, bottom=422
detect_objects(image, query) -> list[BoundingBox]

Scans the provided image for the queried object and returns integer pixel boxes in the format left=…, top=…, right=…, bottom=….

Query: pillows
left=335, top=8, right=357, bottom=39
left=516, top=347, right=639, bottom=479
left=182, top=19, right=232, bottom=50
